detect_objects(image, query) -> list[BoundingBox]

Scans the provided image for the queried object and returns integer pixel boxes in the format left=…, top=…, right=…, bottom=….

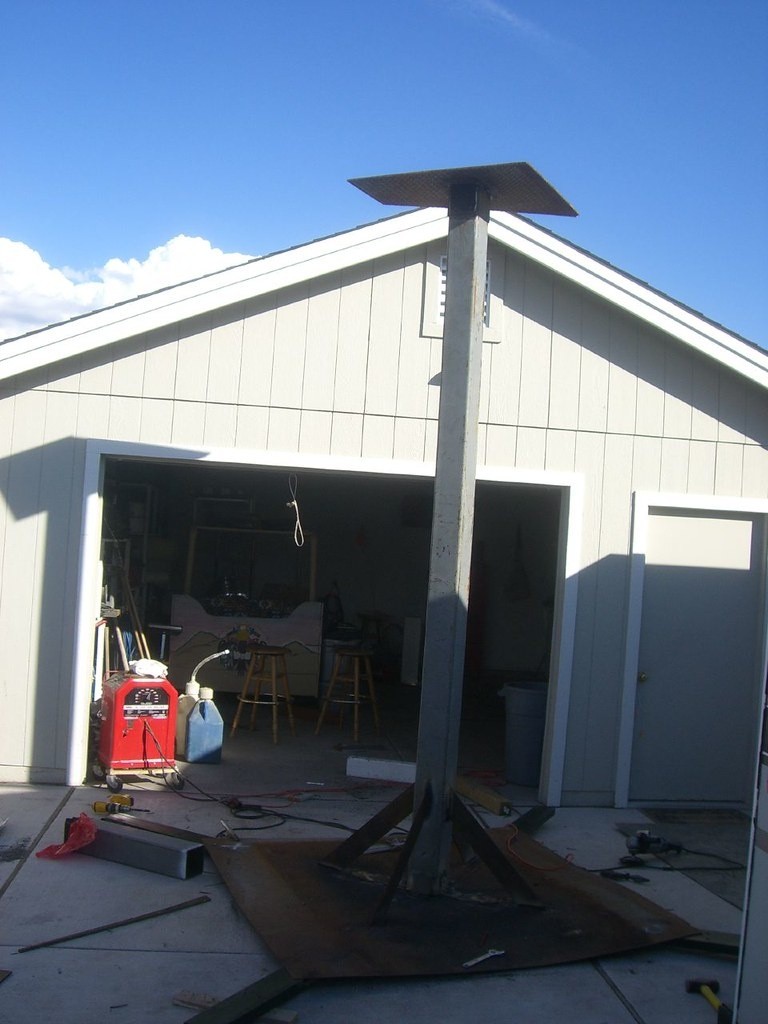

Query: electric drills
left=91, top=793, right=151, bottom=813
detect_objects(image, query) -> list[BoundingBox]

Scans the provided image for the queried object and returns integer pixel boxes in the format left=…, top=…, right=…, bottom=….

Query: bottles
left=184, top=687, right=225, bottom=764
left=175, top=681, right=201, bottom=758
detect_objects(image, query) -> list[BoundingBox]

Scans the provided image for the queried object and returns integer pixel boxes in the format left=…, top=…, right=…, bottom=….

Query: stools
left=229, top=647, right=294, bottom=743
left=315, top=648, right=380, bottom=741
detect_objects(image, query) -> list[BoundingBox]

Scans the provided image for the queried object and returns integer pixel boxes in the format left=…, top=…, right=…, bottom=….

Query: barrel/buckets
left=502, top=680, right=548, bottom=788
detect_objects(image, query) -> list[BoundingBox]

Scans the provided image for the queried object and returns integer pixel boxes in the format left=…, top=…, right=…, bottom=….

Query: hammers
left=685, top=977, right=733, bottom=1024
